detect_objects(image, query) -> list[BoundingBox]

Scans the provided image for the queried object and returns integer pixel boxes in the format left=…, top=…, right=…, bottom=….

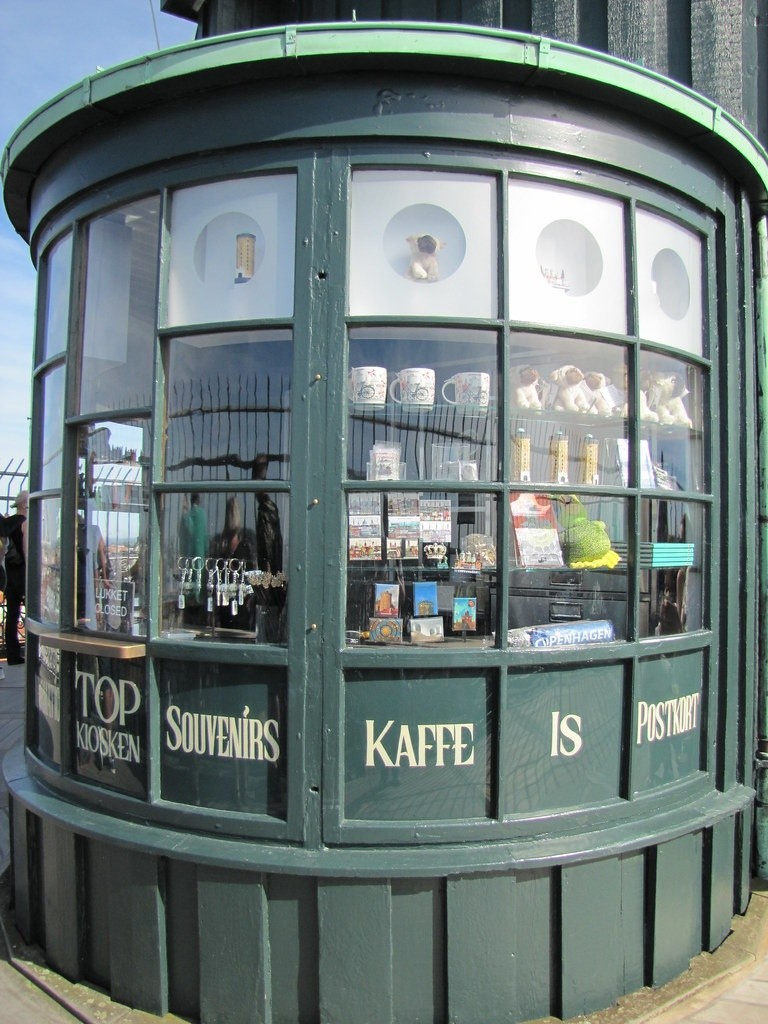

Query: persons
left=659, top=597, right=683, bottom=635
left=52, top=512, right=112, bottom=631
left=178, top=452, right=284, bottom=645
left=0, top=490, right=28, bottom=678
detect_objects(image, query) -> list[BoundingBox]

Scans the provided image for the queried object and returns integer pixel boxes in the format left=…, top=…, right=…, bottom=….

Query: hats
left=9, top=490, right=28, bottom=508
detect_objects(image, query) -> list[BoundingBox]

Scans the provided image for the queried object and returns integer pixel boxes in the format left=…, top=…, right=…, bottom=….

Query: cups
left=389, top=368, right=436, bottom=412
left=349, top=366, right=387, bottom=410
left=432, top=442, right=470, bottom=479
left=442, top=372, right=489, bottom=415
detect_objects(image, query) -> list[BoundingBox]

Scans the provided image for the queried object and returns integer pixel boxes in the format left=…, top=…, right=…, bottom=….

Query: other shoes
left=7, top=657, right=25, bottom=665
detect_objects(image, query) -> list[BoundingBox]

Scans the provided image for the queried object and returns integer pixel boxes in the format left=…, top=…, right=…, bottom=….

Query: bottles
left=549, top=431, right=568, bottom=483
left=580, top=434, right=598, bottom=484
left=510, top=428, right=531, bottom=482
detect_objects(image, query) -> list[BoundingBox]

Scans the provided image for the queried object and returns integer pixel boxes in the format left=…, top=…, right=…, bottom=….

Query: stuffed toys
left=405, top=232, right=444, bottom=281
left=510, top=361, right=692, bottom=428
left=549, top=494, right=618, bottom=570
